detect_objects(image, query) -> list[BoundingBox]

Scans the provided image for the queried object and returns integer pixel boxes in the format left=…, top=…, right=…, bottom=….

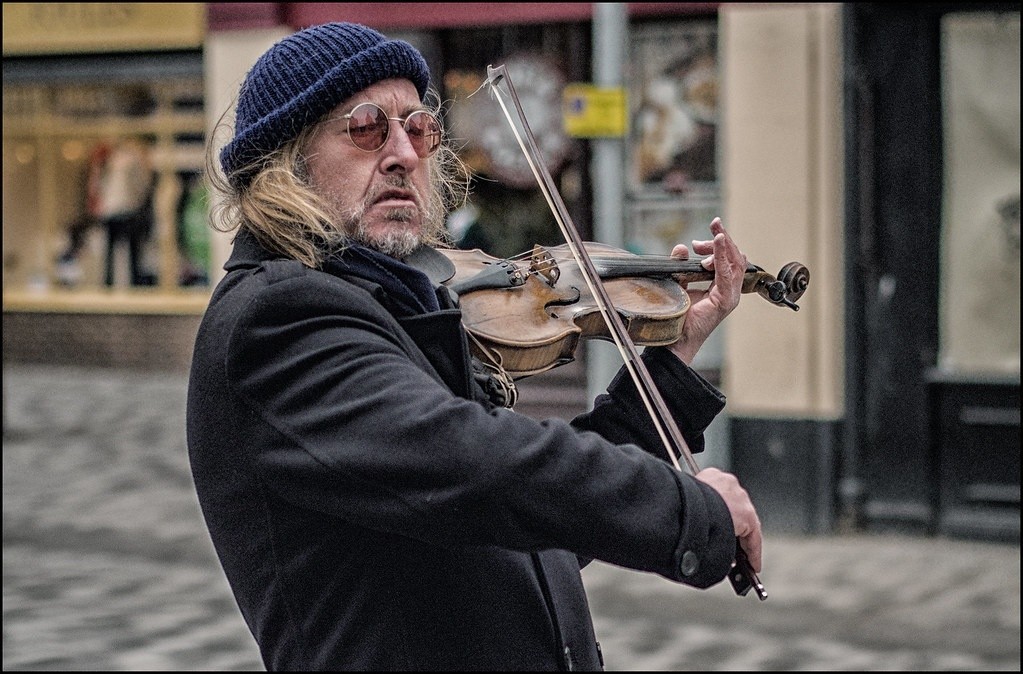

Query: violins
left=435, top=242, right=812, bottom=377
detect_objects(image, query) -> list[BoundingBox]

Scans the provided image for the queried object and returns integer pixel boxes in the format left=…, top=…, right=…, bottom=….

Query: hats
left=216, top=21, right=430, bottom=184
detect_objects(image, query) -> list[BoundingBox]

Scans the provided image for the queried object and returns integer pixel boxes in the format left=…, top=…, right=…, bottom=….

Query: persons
left=186, top=21, right=763, bottom=674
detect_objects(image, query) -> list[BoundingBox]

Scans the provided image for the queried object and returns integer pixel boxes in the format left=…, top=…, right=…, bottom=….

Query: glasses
left=318, top=102, right=445, bottom=159
